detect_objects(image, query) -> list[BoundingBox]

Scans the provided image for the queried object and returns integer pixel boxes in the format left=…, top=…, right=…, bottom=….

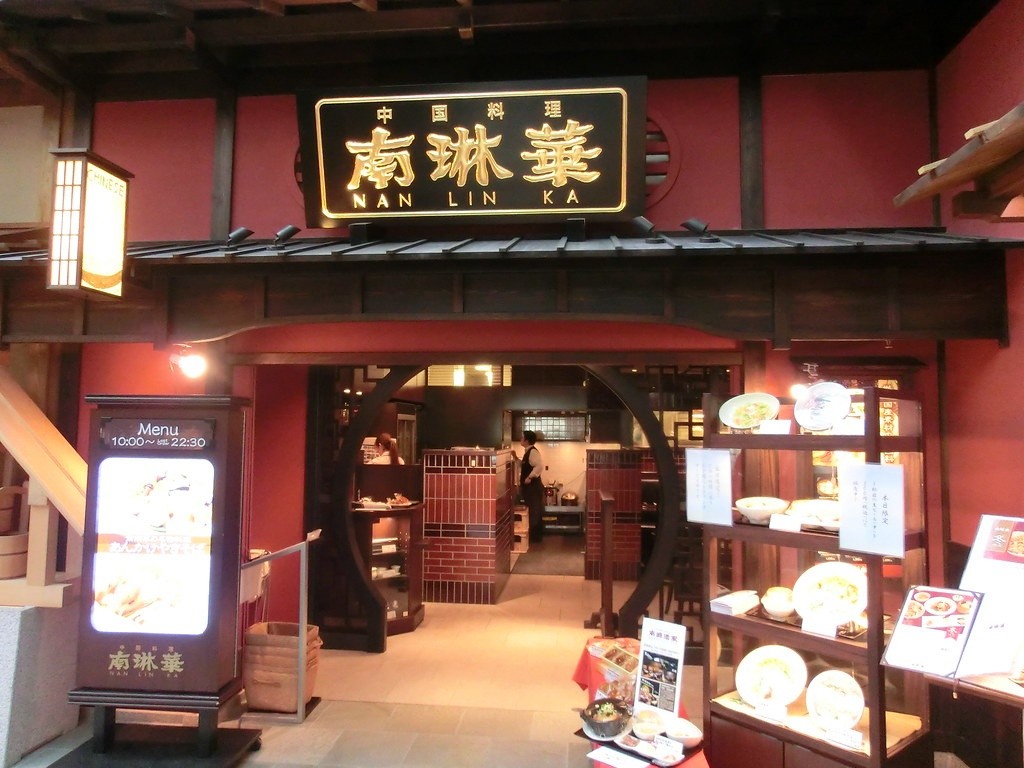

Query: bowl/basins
left=761, top=587, right=796, bottom=617
left=664, top=718, right=703, bottom=748
left=583, top=698, right=625, bottom=735
left=632, top=711, right=665, bottom=741
left=734, top=496, right=790, bottom=526
left=914, top=592, right=930, bottom=602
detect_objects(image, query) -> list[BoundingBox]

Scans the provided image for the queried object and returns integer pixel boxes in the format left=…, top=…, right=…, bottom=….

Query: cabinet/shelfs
left=923, top=673, right=1024, bottom=768
left=351, top=501, right=434, bottom=638
left=376, top=401, right=417, bottom=465
left=702, top=389, right=936, bottom=768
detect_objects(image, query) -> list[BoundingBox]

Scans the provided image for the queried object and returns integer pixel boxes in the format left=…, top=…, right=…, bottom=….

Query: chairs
left=655, top=534, right=761, bottom=642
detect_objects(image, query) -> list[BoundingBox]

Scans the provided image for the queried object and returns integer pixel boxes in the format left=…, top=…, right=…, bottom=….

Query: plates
left=581, top=718, right=633, bottom=742
left=924, top=597, right=957, bottom=616
left=734, top=644, right=808, bottom=710
left=903, top=602, right=925, bottom=619
left=792, top=562, right=868, bottom=627
left=806, top=671, right=864, bottom=733
left=794, top=382, right=852, bottom=433
left=719, top=393, right=781, bottom=429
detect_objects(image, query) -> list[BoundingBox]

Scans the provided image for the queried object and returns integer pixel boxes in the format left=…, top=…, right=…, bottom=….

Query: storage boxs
left=241, top=622, right=323, bottom=714
left=510, top=504, right=530, bottom=554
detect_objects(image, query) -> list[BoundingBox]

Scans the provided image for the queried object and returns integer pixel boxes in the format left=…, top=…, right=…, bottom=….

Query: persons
left=511, top=431, right=545, bottom=542
left=368, top=433, right=404, bottom=587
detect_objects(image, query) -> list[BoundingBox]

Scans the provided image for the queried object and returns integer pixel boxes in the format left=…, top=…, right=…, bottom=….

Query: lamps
left=632, top=216, right=665, bottom=243
left=227, top=226, right=255, bottom=247
left=681, top=217, right=721, bottom=243
left=273, top=224, right=303, bottom=246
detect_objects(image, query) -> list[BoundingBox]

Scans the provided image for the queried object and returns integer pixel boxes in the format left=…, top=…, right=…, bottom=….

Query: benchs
left=572, top=637, right=710, bottom=768
